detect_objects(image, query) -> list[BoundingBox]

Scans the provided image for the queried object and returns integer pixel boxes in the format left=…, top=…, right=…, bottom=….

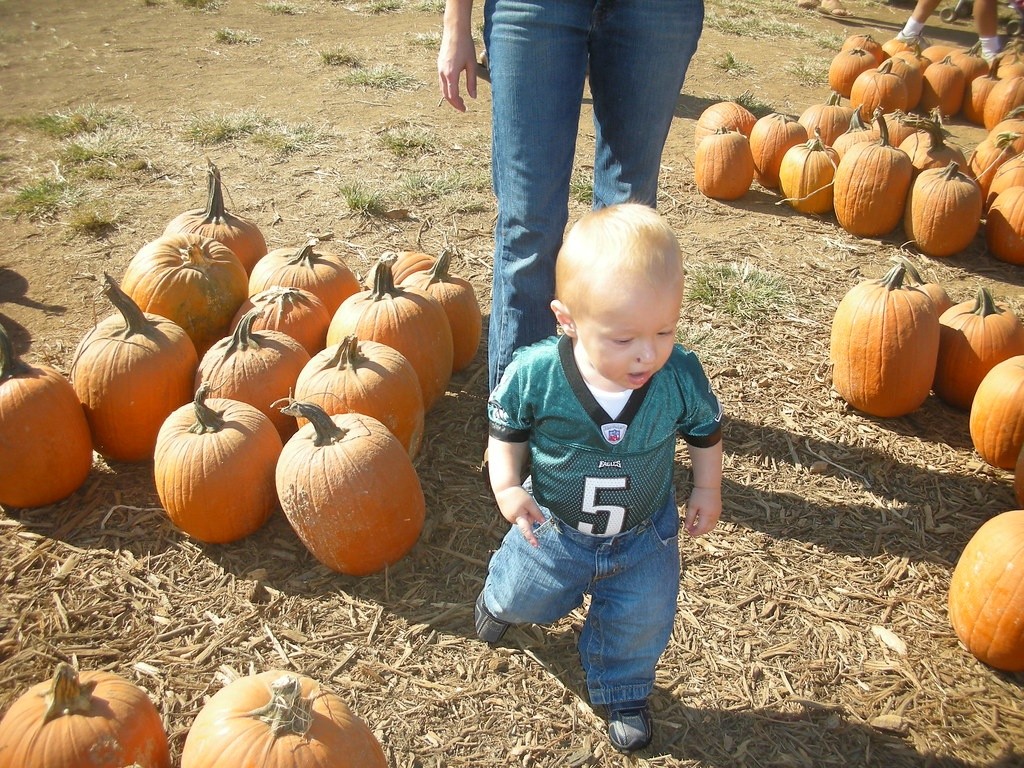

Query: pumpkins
left=829, top=256, right=1024, bottom=674
left=693, top=34, right=1024, bottom=266
left=0, top=158, right=479, bottom=577
left=0, top=661, right=392, bottom=768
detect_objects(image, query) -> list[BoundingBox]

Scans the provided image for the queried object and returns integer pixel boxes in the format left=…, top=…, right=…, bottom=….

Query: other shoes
left=482, top=436, right=530, bottom=474
left=897, top=30, right=927, bottom=51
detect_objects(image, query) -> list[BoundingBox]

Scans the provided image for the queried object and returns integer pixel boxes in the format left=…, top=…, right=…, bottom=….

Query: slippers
left=818, top=6, right=856, bottom=17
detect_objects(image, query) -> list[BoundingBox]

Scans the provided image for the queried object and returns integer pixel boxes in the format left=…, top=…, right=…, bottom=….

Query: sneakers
left=604, top=701, right=653, bottom=749
left=474, top=589, right=512, bottom=643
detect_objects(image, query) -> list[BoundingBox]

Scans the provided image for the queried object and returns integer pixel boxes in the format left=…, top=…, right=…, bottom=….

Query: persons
left=437, top=0, right=706, bottom=504
left=894, top=0, right=1002, bottom=69
left=474, top=200, right=726, bottom=750
